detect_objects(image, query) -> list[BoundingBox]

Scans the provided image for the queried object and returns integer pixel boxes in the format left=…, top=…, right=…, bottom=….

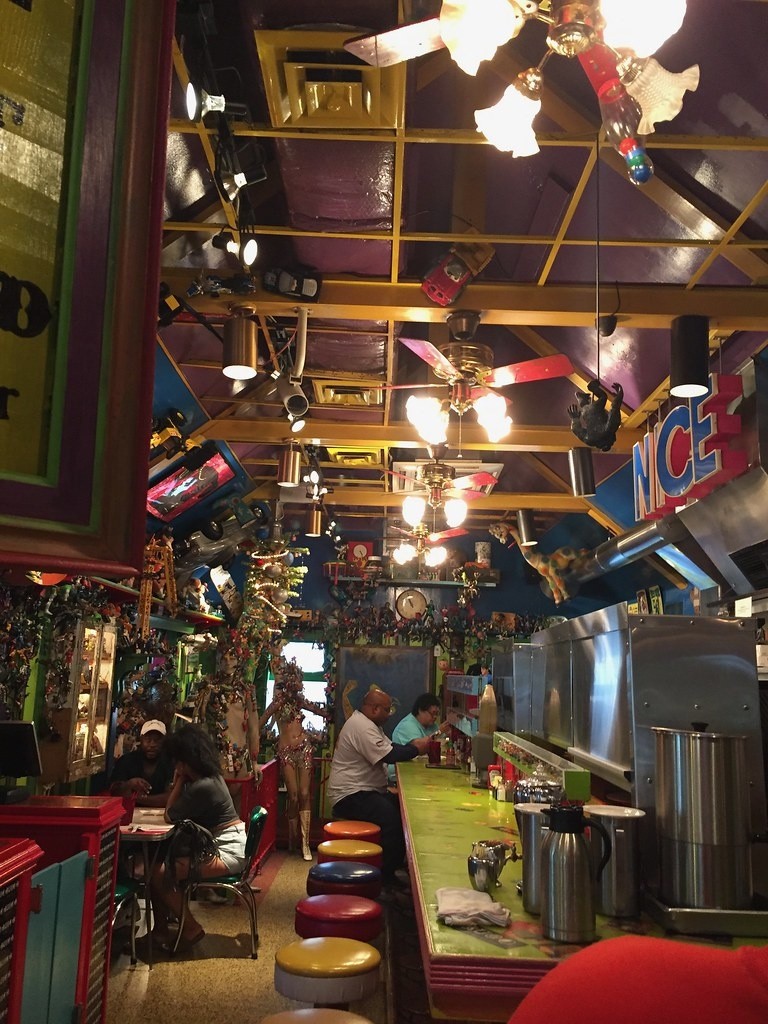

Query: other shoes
left=114, top=898, right=140, bottom=929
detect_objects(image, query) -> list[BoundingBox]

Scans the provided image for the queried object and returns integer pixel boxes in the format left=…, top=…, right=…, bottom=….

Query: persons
left=480, top=665, right=492, bottom=691
left=327, top=689, right=429, bottom=884
left=258, top=661, right=330, bottom=860
left=154, top=723, right=247, bottom=954
left=112, top=719, right=174, bottom=809
left=387, top=693, right=450, bottom=802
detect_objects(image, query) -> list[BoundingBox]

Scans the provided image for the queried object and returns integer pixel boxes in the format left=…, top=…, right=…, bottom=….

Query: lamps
left=185, top=0, right=712, bottom=568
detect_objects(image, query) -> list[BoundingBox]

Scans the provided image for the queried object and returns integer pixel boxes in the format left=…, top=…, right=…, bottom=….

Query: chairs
left=113, top=806, right=267, bottom=966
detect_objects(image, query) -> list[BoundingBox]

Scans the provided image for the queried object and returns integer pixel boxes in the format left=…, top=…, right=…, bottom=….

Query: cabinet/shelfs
left=32, top=606, right=119, bottom=783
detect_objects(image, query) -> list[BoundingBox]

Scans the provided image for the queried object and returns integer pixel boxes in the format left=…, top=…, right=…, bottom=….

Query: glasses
left=427, top=710, right=440, bottom=717
left=378, top=705, right=391, bottom=713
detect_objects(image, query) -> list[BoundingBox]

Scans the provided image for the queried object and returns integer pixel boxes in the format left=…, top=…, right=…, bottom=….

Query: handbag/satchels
left=157, top=818, right=216, bottom=859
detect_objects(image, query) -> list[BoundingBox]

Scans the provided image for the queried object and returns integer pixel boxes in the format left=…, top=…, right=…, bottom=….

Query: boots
left=299, top=809, right=313, bottom=861
left=288, top=816, right=299, bottom=851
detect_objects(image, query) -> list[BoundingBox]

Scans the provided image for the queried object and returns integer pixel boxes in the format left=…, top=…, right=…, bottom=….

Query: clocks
left=396, top=589, right=428, bottom=619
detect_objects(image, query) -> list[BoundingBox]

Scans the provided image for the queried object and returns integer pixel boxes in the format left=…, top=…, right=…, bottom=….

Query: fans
left=375, top=520, right=468, bottom=550
left=360, top=310, right=574, bottom=407
left=379, top=445, right=498, bottom=503
left=343, top=12, right=446, bottom=66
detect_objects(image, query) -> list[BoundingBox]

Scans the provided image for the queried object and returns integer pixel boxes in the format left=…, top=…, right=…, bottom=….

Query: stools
left=261, top=809, right=384, bottom=1024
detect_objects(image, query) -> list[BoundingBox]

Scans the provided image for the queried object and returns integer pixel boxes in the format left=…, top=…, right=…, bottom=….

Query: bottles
left=514, top=762, right=567, bottom=838
left=446, top=738, right=477, bottom=773
left=488, top=764, right=513, bottom=802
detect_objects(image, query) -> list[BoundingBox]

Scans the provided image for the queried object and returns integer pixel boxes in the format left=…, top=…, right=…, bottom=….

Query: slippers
left=135, top=933, right=161, bottom=948
left=164, top=931, right=205, bottom=952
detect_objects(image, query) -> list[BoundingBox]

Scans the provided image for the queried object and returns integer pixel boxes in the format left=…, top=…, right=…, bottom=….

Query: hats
left=140, top=720, right=166, bottom=736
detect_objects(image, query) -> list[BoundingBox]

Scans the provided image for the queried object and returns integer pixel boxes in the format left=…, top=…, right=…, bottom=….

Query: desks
left=118, top=805, right=178, bottom=970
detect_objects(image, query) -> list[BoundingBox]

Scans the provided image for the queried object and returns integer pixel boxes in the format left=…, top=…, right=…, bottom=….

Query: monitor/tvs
left=0, top=718, right=43, bottom=804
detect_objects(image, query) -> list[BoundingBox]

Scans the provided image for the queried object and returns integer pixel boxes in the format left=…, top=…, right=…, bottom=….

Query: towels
left=433, top=886, right=511, bottom=927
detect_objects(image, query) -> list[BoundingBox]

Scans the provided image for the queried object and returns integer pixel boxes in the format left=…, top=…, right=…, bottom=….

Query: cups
left=428, top=741, right=441, bottom=764
left=466, top=839, right=506, bottom=900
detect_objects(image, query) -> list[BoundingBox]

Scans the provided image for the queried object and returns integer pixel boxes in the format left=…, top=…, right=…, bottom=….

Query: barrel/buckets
left=651, top=720, right=764, bottom=909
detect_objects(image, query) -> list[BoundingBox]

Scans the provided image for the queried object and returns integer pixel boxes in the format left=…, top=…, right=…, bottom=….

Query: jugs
left=582, top=804, right=648, bottom=918
left=513, top=802, right=552, bottom=915
left=540, top=804, right=613, bottom=945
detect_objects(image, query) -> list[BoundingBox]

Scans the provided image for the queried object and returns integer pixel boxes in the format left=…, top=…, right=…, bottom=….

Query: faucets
left=499, top=842, right=523, bottom=865
